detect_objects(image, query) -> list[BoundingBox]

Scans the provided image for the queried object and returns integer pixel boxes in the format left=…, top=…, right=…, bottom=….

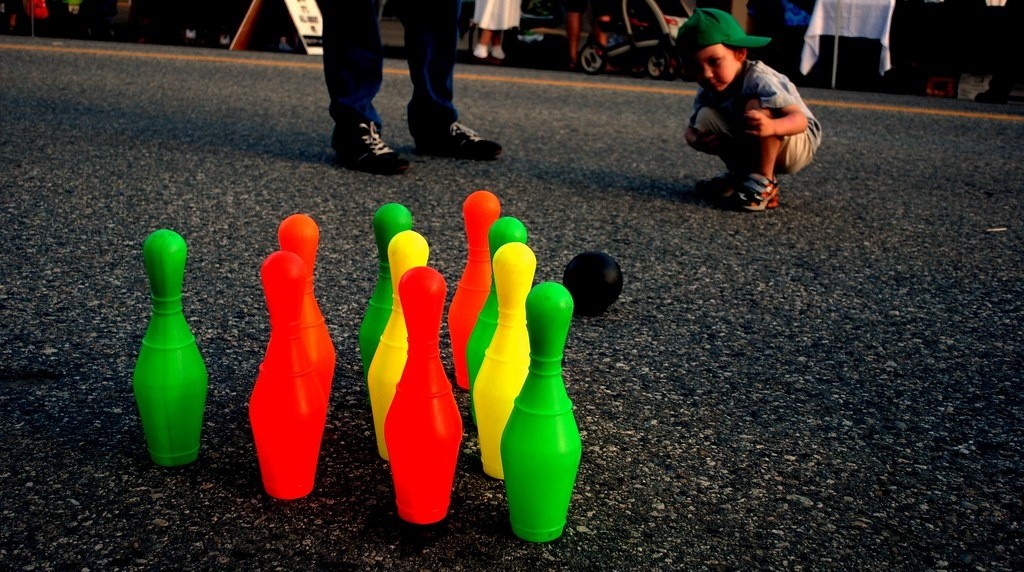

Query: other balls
left=564, top=251, right=622, bottom=313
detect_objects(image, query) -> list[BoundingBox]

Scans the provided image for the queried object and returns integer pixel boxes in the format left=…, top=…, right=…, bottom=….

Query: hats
left=675, top=6, right=772, bottom=61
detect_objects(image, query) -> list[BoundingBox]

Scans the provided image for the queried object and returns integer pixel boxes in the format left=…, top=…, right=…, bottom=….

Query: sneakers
left=694, top=172, right=741, bottom=207
left=331, top=121, right=409, bottom=173
left=410, top=115, right=502, bottom=158
left=715, top=172, right=780, bottom=210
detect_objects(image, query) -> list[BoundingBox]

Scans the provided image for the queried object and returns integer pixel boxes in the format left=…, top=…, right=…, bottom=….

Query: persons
left=676, top=7, right=821, bottom=210
left=562, top=0, right=620, bottom=74
left=316, top=0, right=502, bottom=174
left=472, top=0, right=522, bottom=59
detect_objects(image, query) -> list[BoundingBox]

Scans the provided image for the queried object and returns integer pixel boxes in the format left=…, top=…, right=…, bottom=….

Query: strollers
left=579, top=1, right=695, bottom=81
